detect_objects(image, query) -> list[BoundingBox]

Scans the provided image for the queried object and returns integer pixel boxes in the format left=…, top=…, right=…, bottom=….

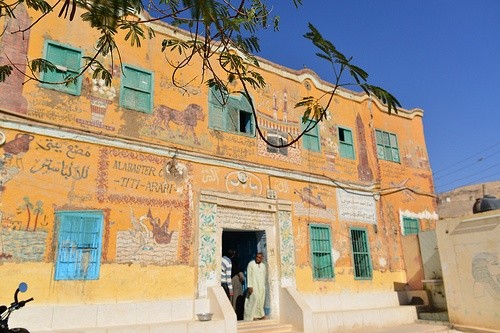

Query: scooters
left=0, top=282, right=34, bottom=333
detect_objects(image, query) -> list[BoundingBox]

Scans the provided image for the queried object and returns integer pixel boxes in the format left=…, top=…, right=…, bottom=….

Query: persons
left=222, top=248, right=266, bottom=321
